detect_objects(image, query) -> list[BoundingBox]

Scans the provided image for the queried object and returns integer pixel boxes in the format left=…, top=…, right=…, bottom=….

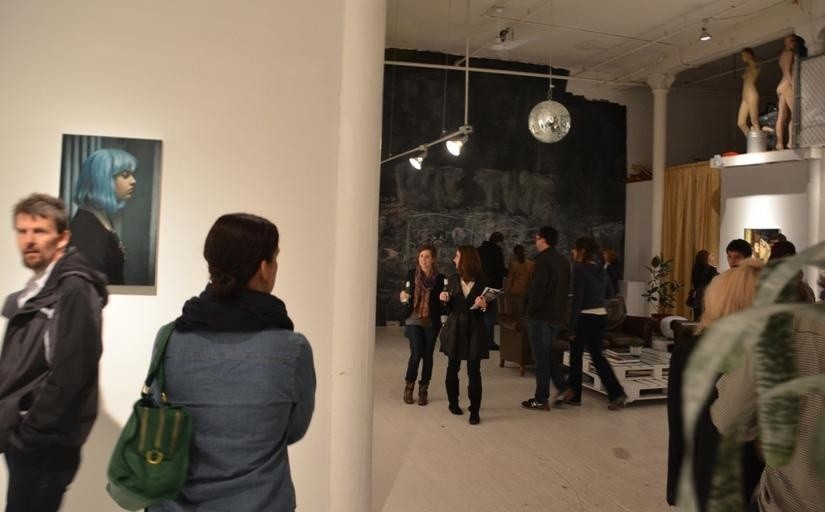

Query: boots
left=404, top=381, right=414, bottom=404
left=445, top=379, right=463, bottom=415
left=467, top=385, right=482, bottom=424
left=418, top=380, right=428, bottom=405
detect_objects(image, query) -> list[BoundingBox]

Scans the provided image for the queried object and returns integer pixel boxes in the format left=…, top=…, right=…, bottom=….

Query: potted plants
left=641, top=252, right=685, bottom=322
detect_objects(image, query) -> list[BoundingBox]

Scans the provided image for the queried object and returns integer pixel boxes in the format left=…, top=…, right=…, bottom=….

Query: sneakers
left=521, top=383, right=581, bottom=411
left=607, top=393, right=627, bottom=411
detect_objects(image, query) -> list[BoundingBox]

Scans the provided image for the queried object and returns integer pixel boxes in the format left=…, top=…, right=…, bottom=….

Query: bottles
left=405, top=281, right=411, bottom=294
left=443, top=278, right=449, bottom=293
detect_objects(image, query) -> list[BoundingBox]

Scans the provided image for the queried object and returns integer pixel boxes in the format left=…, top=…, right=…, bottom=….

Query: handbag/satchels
left=605, top=297, right=627, bottom=332
left=106, top=396, right=192, bottom=511
left=686, top=288, right=694, bottom=306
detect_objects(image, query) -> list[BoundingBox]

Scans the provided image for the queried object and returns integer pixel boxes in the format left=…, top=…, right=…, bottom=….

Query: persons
left=521, top=228, right=627, bottom=411
left=477, top=231, right=534, bottom=350
left=438, top=245, right=490, bottom=425
left=1, top=193, right=109, bottom=511
left=68, top=148, right=138, bottom=285
left=686, top=232, right=824, bottom=512
left=399, top=245, right=448, bottom=406
left=143, top=213, right=316, bottom=512
left=736, top=47, right=761, bottom=139
left=775, top=35, right=802, bottom=149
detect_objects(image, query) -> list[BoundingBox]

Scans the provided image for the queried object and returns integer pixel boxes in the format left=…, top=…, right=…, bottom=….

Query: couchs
left=494, top=292, right=651, bottom=377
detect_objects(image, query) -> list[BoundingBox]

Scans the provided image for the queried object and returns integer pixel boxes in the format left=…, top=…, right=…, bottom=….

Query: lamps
left=445, top=134, right=470, bottom=157
left=409, top=150, right=428, bottom=171
left=699, top=28, right=712, bottom=41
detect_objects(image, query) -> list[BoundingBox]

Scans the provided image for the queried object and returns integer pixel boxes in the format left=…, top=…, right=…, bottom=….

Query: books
left=470, top=287, right=505, bottom=310
